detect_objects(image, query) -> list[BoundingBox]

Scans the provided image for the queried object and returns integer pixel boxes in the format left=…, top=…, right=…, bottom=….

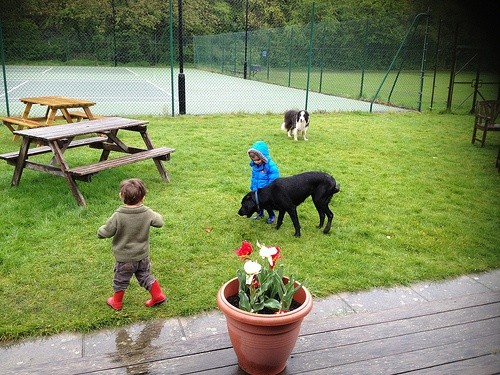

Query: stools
left=68, top=111, right=106, bottom=138
left=64, top=146, right=176, bottom=177
left=0, top=137, right=109, bottom=161
left=0, top=117, right=50, bottom=149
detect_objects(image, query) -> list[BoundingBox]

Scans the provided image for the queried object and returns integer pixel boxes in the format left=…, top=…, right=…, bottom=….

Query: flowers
left=234, top=239, right=306, bottom=311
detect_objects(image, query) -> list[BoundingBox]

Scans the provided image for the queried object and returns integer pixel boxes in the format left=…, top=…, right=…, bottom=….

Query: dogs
left=281, top=110, right=310, bottom=142
left=238, top=171, right=340, bottom=238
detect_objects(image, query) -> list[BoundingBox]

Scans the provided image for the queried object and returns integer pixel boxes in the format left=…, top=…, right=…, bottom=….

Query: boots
left=256, top=209, right=264, bottom=217
left=145, top=281, right=166, bottom=307
left=267, top=211, right=276, bottom=224
left=106, top=290, right=125, bottom=311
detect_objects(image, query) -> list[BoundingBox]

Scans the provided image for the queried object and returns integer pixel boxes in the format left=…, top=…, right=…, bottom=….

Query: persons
left=247, top=140, right=280, bottom=224
left=97, top=178, right=167, bottom=311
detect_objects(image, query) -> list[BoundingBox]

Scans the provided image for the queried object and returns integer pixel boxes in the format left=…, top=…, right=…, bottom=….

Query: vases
left=216, top=275, right=311, bottom=375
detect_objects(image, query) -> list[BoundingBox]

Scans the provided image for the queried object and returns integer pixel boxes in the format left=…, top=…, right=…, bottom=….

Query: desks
left=13, top=96, right=95, bottom=139
left=11, top=116, right=170, bottom=205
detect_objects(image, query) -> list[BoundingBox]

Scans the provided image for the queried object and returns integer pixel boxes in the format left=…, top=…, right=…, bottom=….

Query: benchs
left=472, top=100, right=500, bottom=147
